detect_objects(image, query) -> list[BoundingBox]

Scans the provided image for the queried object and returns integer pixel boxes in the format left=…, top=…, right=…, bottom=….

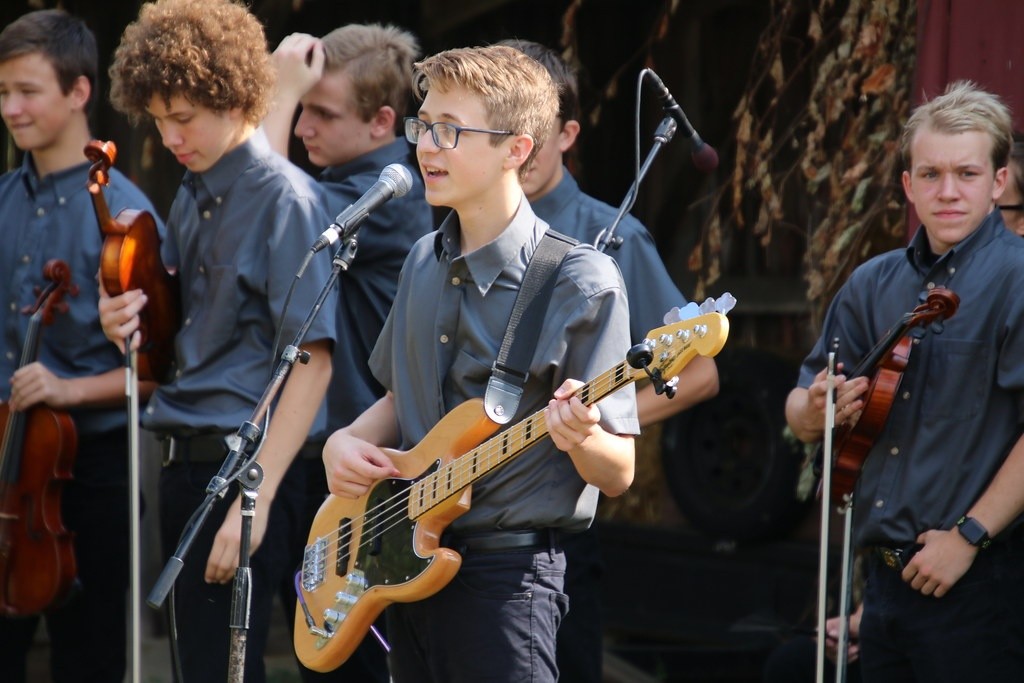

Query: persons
left=492, top=38, right=720, bottom=682
left=784, top=83, right=1024, bottom=683
left=263, top=22, right=431, bottom=442
left=318, top=46, right=641, bottom=683
left=0, top=9, right=169, bottom=683
left=92, top=0, right=396, bottom=683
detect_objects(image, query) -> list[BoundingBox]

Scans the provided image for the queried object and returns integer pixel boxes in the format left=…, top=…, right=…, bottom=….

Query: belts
left=159, top=436, right=323, bottom=467
left=444, top=529, right=547, bottom=551
left=878, top=545, right=919, bottom=567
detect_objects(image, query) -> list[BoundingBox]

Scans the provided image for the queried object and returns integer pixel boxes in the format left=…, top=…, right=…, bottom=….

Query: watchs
left=957, top=516, right=990, bottom=549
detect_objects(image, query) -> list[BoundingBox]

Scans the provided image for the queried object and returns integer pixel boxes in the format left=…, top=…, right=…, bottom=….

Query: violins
left=85, top=133, right=182, bottom=386
left=813, top=280, right=964, bottom=508
left=0, top=256, right=83, bottom=621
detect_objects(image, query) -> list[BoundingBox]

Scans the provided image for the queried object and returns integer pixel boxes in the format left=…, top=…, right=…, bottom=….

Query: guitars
left=290, top=286, right=741, bottom=675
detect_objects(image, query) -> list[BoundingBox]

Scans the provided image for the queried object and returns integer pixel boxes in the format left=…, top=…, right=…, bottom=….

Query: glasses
left=403, top=116, right=517, bottom=149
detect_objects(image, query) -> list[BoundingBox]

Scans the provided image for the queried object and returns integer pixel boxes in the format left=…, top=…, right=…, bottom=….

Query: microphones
left=313, top=163, right=413, bottom=253
left=641, top=66, right=721, bottom=172
